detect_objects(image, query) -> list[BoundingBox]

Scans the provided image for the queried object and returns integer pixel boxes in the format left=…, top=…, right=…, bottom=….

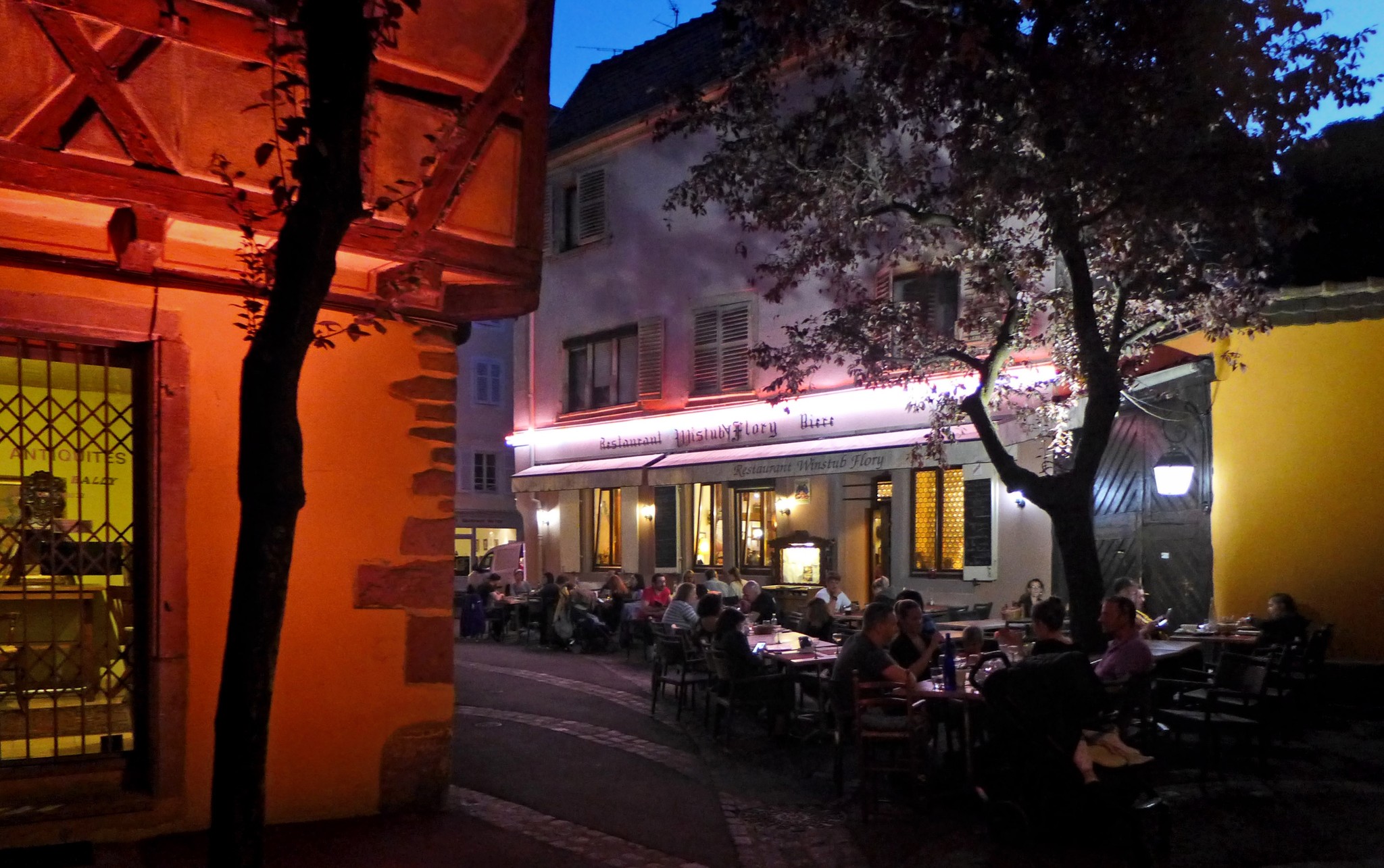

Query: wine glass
left=832, top=633, right=843, bottom=654
left=810, top=637, right=820, bottom=659
left=967, top=654, right=979, bottom=686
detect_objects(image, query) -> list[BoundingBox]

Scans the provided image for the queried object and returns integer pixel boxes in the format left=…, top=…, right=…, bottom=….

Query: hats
left=487, top=573, right=500, bottom=580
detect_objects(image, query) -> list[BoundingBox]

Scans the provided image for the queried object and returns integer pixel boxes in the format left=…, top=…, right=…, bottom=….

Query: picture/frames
left=0, top=475, right=61, bottom=584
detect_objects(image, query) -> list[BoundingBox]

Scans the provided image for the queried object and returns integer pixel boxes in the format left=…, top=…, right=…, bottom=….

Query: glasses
left=1029, top=586, right=1040, bottom=588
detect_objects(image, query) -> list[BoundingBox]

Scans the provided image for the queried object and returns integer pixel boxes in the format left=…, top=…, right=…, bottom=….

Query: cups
left=930, top=667, right=943, bottom=690
left=1008, top=646, right=1019, bottom=662
left=762, top=620, right=770, bottom=626
left=843, top=606, right=852, bottom=616
left=798, top=636, right=809, bottom=649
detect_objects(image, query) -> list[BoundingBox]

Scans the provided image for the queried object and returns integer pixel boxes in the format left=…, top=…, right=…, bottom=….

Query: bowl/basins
left=1217, top=622, right=1241, bottom=631
left=1180, top=624, right=1198, bottom=632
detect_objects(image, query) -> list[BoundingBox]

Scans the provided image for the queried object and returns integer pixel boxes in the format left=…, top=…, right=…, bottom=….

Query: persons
left=1017, top=578, right=1044, bottom=618
left=461, top=564, right=945, bottom=731
left=1091, top=594, right=1152, bottom=695
left=1030, top=595, right=1108, bottom=702
left=957, top=625, right=986, bottom=667
left=1236, top=592, right=1309, bottom=666
left=1112, top=576, right=1208, bottom=688
left=828, top=601, right=938, bottom=790
left=993, top=620, right=1024, bottom=656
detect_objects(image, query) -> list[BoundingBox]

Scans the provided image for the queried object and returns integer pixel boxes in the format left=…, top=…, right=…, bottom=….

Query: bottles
left=771, top=614, right=777, bottom=633
left=943, top=633, right=956, bottom=690
left=1208, top=597, right=1218, bottom=625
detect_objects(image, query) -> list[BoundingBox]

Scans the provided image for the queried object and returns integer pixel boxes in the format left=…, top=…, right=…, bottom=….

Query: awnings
left=508, top=454, right=665, bottom=493
left=647, top=407, right=997, bottom=486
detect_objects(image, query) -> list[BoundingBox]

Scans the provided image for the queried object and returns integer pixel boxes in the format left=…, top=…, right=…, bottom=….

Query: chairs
left=0, top=612, right=28, bottom=714
left=482, top=582, right=1336, bottom=823
left=108, top=585, right=135, bottom=643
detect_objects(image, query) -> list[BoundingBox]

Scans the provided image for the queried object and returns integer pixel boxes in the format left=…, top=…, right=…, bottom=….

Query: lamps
left=774, top=497, right=791, bottom=516
left=1152, top=415, right=1197, bottom=495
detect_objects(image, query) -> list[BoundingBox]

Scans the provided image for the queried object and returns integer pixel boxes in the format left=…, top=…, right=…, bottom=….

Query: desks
left=935, top=619, right=1006, bottom=631
left=892, top=667, right=994, bottom=756
left=0, top=584, right=106, bottom=701
left=498, top=596, right=541, bottom=634
left=1169, top=633, right=1259, bottom=662
left=1141, top=636, right=1202, bottom=663
left=622, top=600, right=648, bottom=659
left=833, top=604, right=949, bottom=620
left=769, top=645, right=844, bottom=711
left=747, top=632, right=819, bottom=656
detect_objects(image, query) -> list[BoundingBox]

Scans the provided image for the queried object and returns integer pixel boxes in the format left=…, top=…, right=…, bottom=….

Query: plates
left=1237, top=630, right=1260, bottom=635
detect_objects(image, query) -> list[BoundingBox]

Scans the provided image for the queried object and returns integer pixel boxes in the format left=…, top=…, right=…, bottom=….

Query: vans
left=466, top=540, right=527, bottom=595
left=454, top=555, right=492, bottom=593
